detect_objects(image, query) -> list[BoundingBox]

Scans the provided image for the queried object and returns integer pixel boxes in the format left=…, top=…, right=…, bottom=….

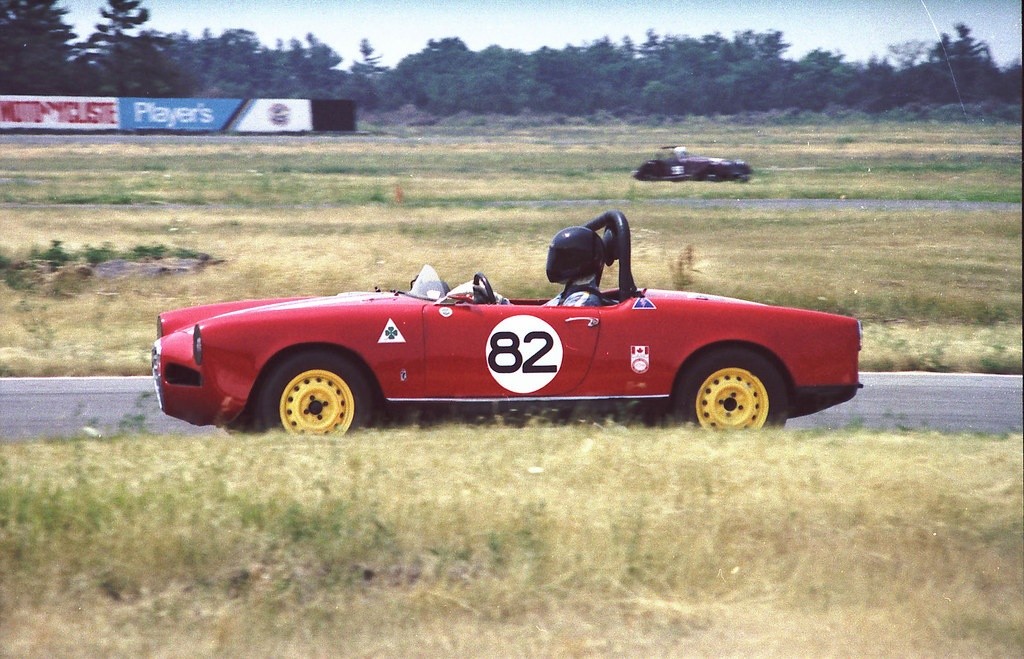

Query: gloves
left=473, top=283, right=510, bottom=305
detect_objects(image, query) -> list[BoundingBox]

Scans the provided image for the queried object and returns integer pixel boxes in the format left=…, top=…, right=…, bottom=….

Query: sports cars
left=630, top=146, right=755, bottom=183
left=150, top=209, right=864, bottom=441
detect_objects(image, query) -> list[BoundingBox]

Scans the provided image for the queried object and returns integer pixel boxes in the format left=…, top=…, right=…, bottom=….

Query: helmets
left=673, top=147, right=688, bottom=160
left=547, top=226, right=604, bottom=282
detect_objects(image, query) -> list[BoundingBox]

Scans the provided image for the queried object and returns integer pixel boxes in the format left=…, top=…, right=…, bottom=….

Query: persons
left=473, top=226, right=606, bottom=307
